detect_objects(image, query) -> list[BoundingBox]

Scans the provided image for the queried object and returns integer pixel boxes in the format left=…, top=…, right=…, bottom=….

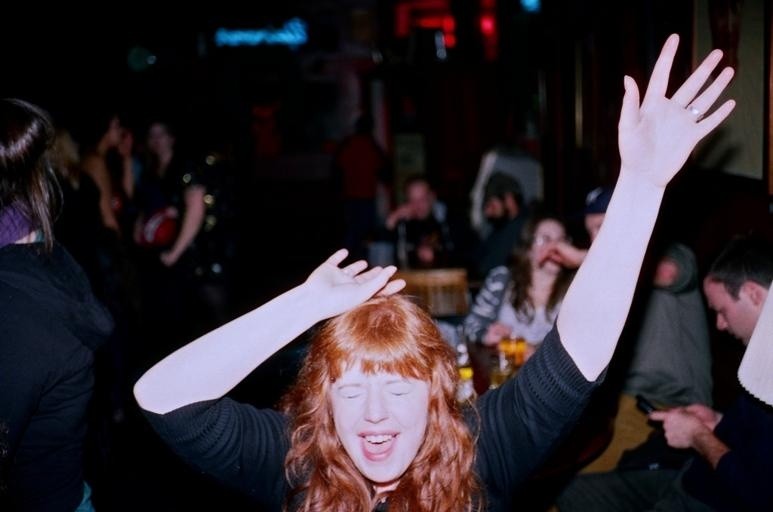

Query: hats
left=584, top=185, right=615, bottom=213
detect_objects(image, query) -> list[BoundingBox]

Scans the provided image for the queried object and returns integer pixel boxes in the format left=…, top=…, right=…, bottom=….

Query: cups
left=498, top=331, right=526, bottom=378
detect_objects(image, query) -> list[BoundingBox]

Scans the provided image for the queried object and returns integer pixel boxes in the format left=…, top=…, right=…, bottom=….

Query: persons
left=0, top=29, right=772, bottom=511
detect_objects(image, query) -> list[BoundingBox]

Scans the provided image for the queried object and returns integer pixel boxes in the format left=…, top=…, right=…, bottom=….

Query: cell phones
left=635, top=393, right=665, bottom=412
